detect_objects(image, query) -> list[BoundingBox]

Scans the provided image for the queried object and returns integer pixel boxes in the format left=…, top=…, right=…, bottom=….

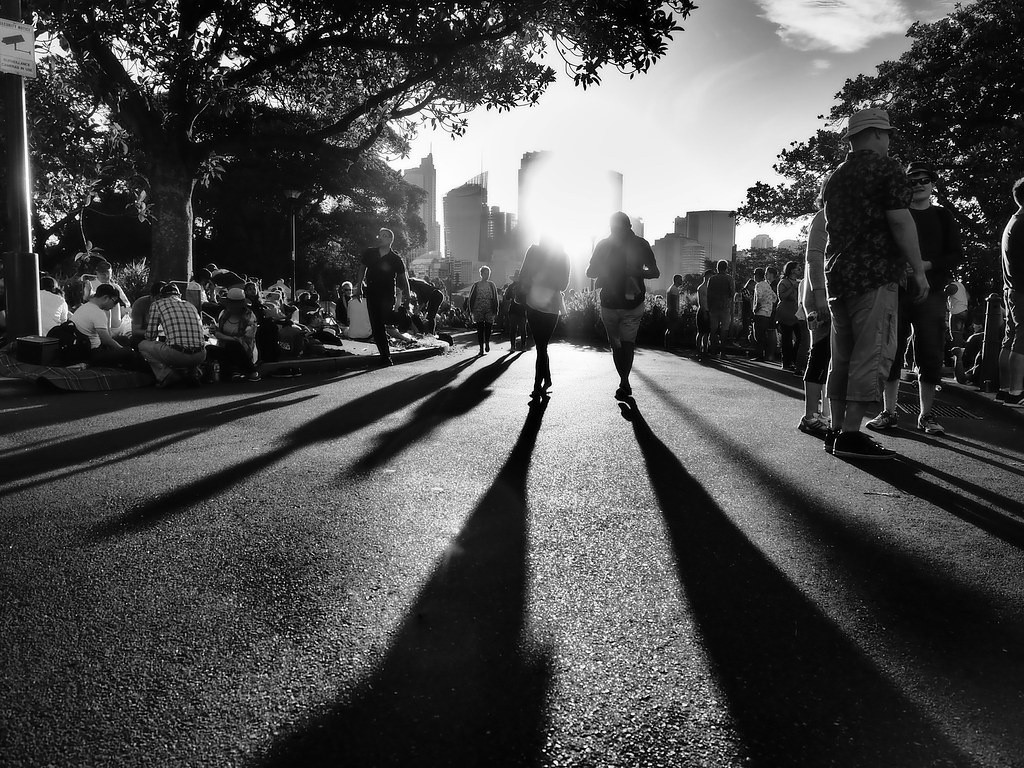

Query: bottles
left=935, top=385, right=942, bottom=392
left=66, top=363, right=89, bottom=371
left=213, top=360, right=219, bottom=382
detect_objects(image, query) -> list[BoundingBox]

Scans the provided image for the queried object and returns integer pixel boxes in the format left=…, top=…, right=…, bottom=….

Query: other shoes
left=533, top=379, right=552, bottom=396
left=695, top=344, right=805, bottom=377
left=521, top=342, right=526, bottom=352
left=994, top=388, right=1024, bottom=408
left=370, top=355, right=393, bottom=367
left=271, top=367, right=302, bottom=377
left=480, top=346, right=484, bottom=356
left=155, top=372, right=174, bottom=389
left=983, top=380, right=994, bottom=393
left=510, top=346, right=515, bottom=352
left=485, top=341, right=489, bottom=352
left=617, top=378, right=631, bottom=399
left=248, top=369, right=261, bottom=380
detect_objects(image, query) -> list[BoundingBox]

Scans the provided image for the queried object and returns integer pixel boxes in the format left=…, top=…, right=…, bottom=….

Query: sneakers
left=865, top=409, right=898, bottom=431
left=823, top=427, right=896, bottom=459
left=798, top=415, right=830, bottom=434
left=916, top=411, right=945, bottom=435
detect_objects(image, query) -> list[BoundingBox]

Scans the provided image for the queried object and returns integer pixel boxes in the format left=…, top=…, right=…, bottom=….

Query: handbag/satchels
left=46, top=321, right=90, bottom=365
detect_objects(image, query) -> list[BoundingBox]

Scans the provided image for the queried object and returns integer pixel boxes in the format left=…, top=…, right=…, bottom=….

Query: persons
left=130, top=280, right=207, bottom=389
left=734, top=261, right=801, bottom=372
left=963, top=333, right=990, bottom=392
left=206, top=263, right=245, bottom=301
left=187, top=268, right=214, bottom=311
left=798, top=109, right=964, bottom=459
left=945, top=276, right=969, bottom=346
left=41, top=259, right=139, bottom=372
left=664, top=259, right=735, bottom=359
left=204, top=274, right=350, bottom=382
left=503, top=212, right=660, bottom=397
left=335, top=227, right=498, bottom=366
left=993, top=175, right=1024, bottom=408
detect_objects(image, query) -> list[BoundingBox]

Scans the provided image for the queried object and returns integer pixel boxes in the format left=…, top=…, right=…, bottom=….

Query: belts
left=172, top=343, right=205, bottom=353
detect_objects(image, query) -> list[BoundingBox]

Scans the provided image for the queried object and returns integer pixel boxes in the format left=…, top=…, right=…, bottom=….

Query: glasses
left=909, top=176, right=930, bottom=187
left=343, top=289, right=352, bottom=291
left=375, top=234, right=389, bottom=239
left=107, top=295, right=119, bottom=306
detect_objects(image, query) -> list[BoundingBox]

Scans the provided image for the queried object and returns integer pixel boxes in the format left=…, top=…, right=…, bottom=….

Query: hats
left=841, top=107, right=898, bottom=144
left=905, top=161, right=938, bottom=183
left=227, top=287, right=244, bottom=300
left=97, top=283, right=126, bottom=307
left=159, top=284, right=181, bottom=295
left=95, top=262, right=111, bottom=273
